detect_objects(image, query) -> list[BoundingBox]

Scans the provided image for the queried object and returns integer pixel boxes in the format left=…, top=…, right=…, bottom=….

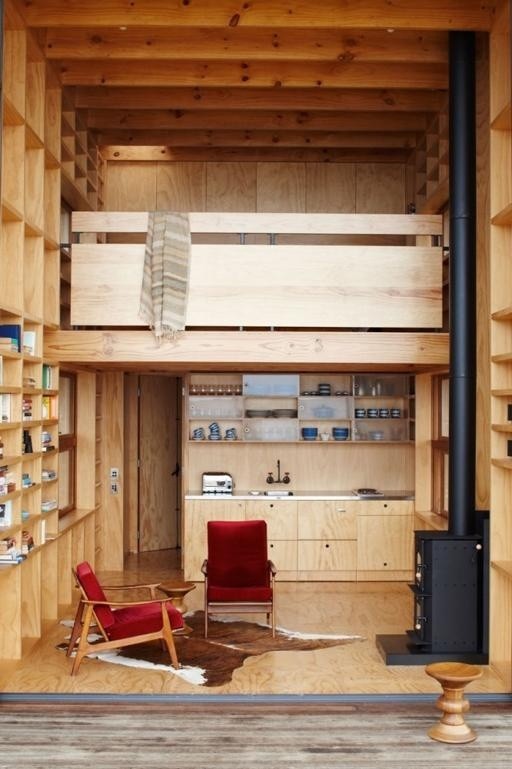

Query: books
left=0, top=322, right=56, bottom=565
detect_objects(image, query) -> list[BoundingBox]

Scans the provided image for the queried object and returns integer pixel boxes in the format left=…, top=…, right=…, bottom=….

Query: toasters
left=201, top=472, right=234, bottom=493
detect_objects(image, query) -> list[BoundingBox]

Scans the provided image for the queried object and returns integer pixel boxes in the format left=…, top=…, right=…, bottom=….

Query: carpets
left=53, top=606, right=363, bottom=690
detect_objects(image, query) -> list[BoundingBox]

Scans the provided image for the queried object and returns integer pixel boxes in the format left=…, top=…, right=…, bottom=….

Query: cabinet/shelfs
left=489, top=15, right=512, bottom=680
left=247, top=499, right=357, bottom=571
left=3, top=68, right=108, bottom=558
left=408, top=101, right=449, bottom=213
left=360, top=501, right=415, bottom=573
left=185, top=371, right=416, bottom=446
left=184, top=500, right=246, bottom=584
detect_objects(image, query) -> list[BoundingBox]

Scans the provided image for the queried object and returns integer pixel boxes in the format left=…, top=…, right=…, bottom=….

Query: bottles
left=190, top=385, right=241, bottom=396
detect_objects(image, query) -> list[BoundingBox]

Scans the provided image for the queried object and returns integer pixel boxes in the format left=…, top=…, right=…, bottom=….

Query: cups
left=319, top=383, right=330, bottom=396
left=371, top=431, right=383, bottom=440
left=356, top=408, right=401, bottom=418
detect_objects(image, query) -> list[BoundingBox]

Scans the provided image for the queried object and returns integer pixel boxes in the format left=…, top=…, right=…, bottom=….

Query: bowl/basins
left=193, top=422, right=237, bottom=441
left=251, top=492, right=260, bottom=496
left=302, top=428, right=348, bottom=441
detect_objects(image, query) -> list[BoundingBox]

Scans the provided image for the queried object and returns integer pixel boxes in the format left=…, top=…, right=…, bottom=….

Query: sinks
left=265, top=491, right=293, bottom=496
left=249, top=490, right=267, bottom=496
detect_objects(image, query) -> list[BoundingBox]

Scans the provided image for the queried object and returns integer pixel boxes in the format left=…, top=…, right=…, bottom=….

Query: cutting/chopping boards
left=353, top=488, right=384, bottom=498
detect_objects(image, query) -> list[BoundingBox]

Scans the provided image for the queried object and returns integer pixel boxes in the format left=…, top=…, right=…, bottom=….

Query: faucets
left=277, top=460, right=281, bottom=482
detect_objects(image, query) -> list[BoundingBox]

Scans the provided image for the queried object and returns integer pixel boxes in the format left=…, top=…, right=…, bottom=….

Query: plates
left=246, top=409, right=297, bottom=418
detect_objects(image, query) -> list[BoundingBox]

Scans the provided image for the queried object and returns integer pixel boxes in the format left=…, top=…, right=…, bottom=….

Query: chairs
left=64, top=560, right=185, bottom=681
left=199, top=517, right=274, bottom=633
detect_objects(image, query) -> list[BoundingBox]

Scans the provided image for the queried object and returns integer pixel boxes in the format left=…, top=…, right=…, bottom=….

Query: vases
left=155, top=579, right=197, bottom=617
left=428, top=660, right=482, bottom=746
